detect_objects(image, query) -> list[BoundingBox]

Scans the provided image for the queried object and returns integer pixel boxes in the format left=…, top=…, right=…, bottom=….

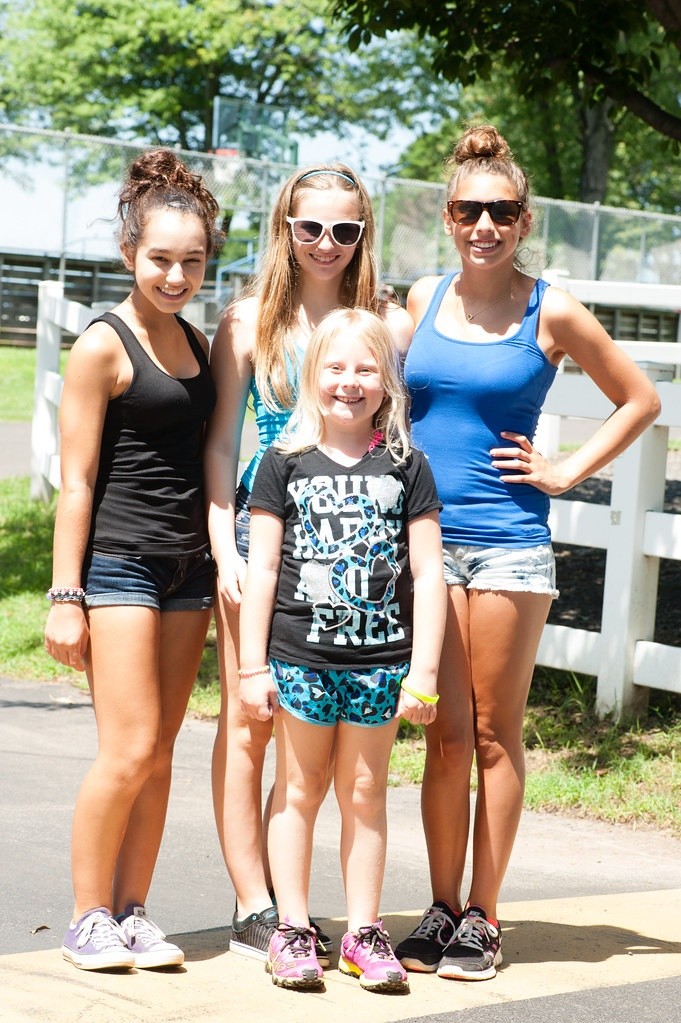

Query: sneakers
left=113, top=902, right=185, bottom=967
left=60, top=906, right=133, bottom=969
left=337, top=921, right=409, bottom=994
left=436, top=906, right=504, bottom=981
left=230, top=889, right=333, bottom=968
left=264, top=924, right=324, bottom=988
left=394, top=902, right=462, bottom=974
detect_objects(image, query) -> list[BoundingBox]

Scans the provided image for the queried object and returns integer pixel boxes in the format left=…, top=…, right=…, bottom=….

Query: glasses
left=447, top=200, right=524, bottom=225
left=286, top=215, right=366, bottom=247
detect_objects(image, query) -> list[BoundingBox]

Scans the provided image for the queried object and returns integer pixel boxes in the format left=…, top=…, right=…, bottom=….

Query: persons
left=405, top=122, right=667, bottom=979
left=44, top=149, right=222, bottom=972
left=212, top=160, right=413, bottom=964
left=234, top=306, right=450, bottom=994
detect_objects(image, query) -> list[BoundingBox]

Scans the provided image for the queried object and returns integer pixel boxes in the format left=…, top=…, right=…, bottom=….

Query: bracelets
left=46, top=587, right=86, bottom=603
left=400, top=676, right=440, bottom=706
left=237, top=659, right=270, bottom=679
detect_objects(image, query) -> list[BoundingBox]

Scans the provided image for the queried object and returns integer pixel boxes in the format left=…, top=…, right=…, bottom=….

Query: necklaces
left=459, top=276, right=517, bottom=323
